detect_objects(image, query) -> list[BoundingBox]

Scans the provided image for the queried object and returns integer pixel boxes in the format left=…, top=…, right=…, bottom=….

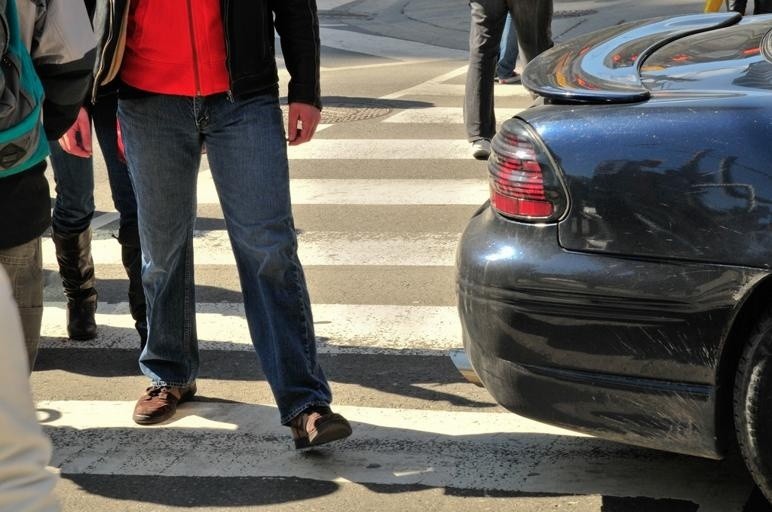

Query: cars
left=449, top=8, right=772, bottom=508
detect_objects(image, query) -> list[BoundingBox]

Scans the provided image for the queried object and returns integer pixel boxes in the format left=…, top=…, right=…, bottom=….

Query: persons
left=728, top=0, right=770, bottom=13
left=90, top=1, right=351, bottom=449
left=465, top=0, right=552, bottom=159
left=19, top=1, right=146, bottom=342
left=0, top=0, right=96, bottom=381
left=0, top=264, right=61, bottom=512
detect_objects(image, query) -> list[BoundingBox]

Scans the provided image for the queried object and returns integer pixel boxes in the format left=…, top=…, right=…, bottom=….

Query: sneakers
left=132, top=381, right=198, bottom=424
left=472, top=140, right=491, bottom=160
left=290, top=405, right=352, bottom=451
left=499, top=72, right=521, bottom=84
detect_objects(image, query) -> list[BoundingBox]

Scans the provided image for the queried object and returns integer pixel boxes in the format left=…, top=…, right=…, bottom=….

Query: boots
left=118, top=236, right=147, bottom=349
left=51, top=224, right=99, bottom=340
